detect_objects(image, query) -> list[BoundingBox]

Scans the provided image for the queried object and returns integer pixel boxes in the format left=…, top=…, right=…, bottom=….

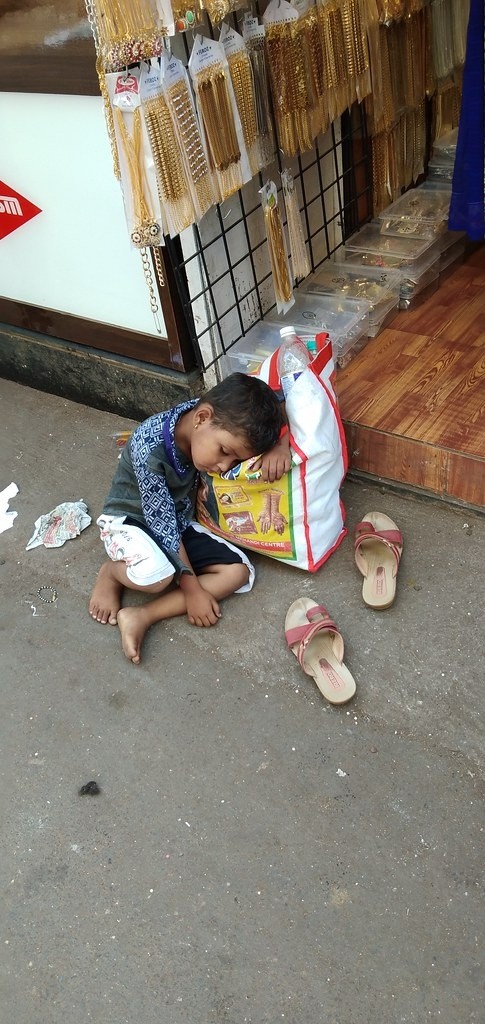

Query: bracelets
left=37, top=586, right=57, bottom=603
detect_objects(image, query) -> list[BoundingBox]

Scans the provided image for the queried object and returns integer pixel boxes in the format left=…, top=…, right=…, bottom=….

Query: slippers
left=355, top=512, right=403, bottom=608
left=285, top=598, right=356, bottom=704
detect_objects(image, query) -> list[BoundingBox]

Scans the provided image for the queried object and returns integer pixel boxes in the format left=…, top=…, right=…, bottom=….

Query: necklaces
left=84, top=0, right=471, bottom=312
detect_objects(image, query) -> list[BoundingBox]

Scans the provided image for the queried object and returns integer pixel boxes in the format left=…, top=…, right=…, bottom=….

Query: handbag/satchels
left=192, top=334, right=349, bottom=572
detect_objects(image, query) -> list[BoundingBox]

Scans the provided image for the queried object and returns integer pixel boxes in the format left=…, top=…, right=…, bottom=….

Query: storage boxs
left=229, top=180, right=468, bottom=392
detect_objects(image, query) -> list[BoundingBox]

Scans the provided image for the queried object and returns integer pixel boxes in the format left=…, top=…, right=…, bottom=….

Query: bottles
left=276, top=325, right=317, bottom=403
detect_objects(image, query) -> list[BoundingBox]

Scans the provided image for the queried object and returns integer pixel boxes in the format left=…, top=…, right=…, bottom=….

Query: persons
left=219, top=493, right=232, bottom=505
left=256, top=493, right=288, bottom=536
left=90, top=372, right=292, bottom=664
